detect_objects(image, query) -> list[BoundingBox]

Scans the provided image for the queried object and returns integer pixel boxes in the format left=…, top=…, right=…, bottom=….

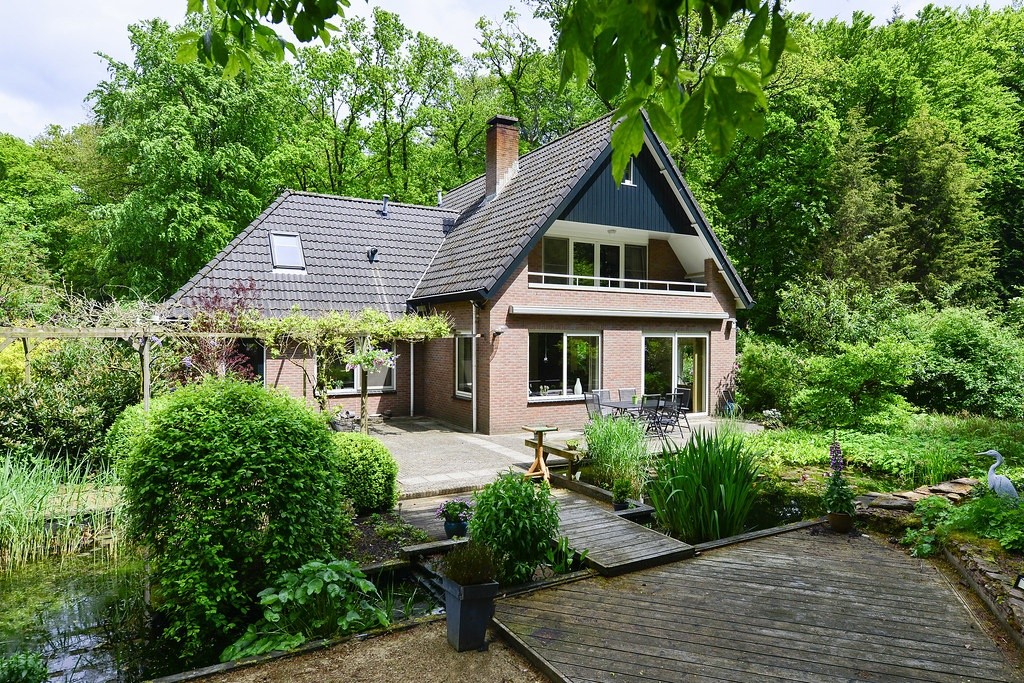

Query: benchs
left=525, top=439, right=585, bottom=481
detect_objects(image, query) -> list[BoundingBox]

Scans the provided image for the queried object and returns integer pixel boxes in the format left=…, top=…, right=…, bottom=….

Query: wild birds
left=974, top=450, right=1019, bottom=501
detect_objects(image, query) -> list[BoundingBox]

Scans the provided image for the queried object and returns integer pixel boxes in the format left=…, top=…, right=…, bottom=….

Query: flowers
left=434, top=496, right=476, bottom=526
left=822, top=430, right=859, bottom=519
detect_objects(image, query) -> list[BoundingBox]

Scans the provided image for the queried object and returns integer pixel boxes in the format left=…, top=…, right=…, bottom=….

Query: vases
left=444, top=520, right=467, bottom=540
left=827, top=512, right=856, bottom=534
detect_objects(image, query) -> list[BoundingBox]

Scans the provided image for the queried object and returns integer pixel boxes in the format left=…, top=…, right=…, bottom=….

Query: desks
left=522, top=423, right=559, bottom=489
left=599, top=399, right=684, bottom=427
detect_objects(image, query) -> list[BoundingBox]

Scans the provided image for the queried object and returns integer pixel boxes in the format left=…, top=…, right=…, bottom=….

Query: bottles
left=575, top=378, right=582, bottom=395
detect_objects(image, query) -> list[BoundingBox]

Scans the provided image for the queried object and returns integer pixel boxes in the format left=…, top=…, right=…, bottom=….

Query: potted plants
left=467, top=466, right=589, bottom=586
left=425, top=533, right=512, bottom=654
left=566, top=439, right=580, bottom=450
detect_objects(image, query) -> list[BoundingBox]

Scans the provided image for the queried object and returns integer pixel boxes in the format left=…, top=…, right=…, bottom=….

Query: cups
left=632, top=398, right=637, bottom=404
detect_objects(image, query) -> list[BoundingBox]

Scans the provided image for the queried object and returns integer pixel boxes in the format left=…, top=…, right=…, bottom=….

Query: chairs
left=585, top=388, right=692, bottom=440
